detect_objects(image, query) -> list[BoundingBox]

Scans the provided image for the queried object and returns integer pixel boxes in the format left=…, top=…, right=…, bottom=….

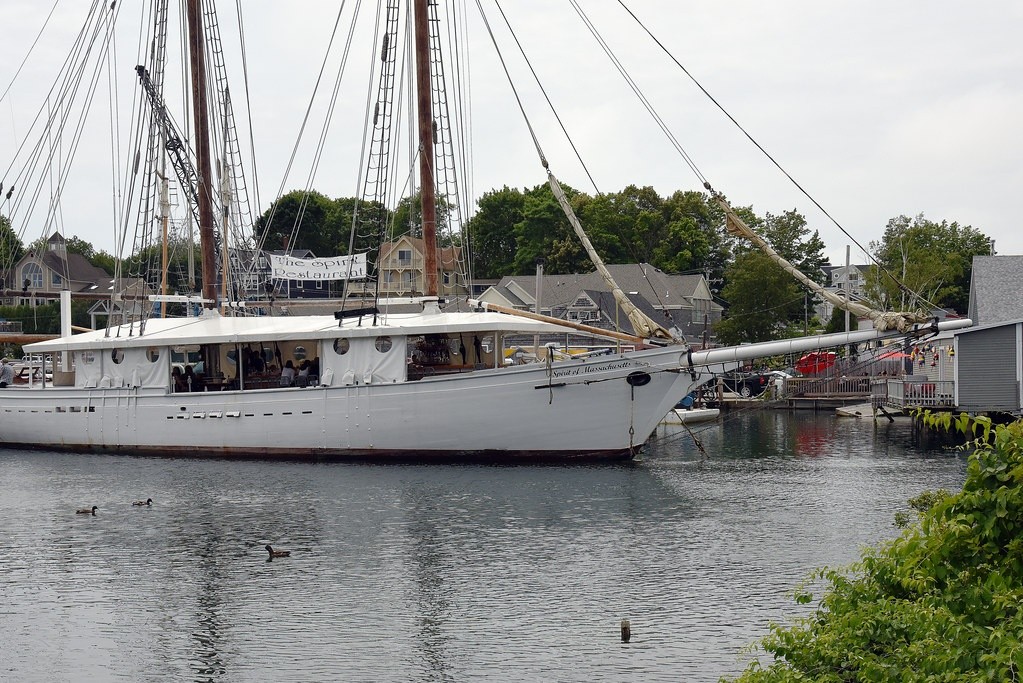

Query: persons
left=172, top=365, right=205, bottom=393
left=247, top=351, right=264, bottom=372
left=298, top=359, right=312, bottom=378
left=280, top=359, right=295, bottom=384
left=0, top=357, right=15, bottom=389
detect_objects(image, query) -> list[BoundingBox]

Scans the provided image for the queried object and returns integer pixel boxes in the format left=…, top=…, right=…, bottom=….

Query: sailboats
left=0, top=0, right=973, bottom=466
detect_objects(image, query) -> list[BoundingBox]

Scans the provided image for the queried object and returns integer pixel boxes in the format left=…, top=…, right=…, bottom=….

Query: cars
left=707, top=370, right=771, bottom=397
left=757, top=370, right=792, bottom=390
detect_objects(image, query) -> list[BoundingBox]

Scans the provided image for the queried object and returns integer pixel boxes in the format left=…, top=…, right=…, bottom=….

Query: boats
left=656, top=394, right=720, bottom=424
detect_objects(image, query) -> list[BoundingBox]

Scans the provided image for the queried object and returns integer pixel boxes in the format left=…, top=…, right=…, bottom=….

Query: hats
left=1, top=358, right=8, bottom=363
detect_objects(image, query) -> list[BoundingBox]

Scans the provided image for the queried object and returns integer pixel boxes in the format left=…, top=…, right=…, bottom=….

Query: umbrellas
left=794, top=352, right=836, bottom=373
